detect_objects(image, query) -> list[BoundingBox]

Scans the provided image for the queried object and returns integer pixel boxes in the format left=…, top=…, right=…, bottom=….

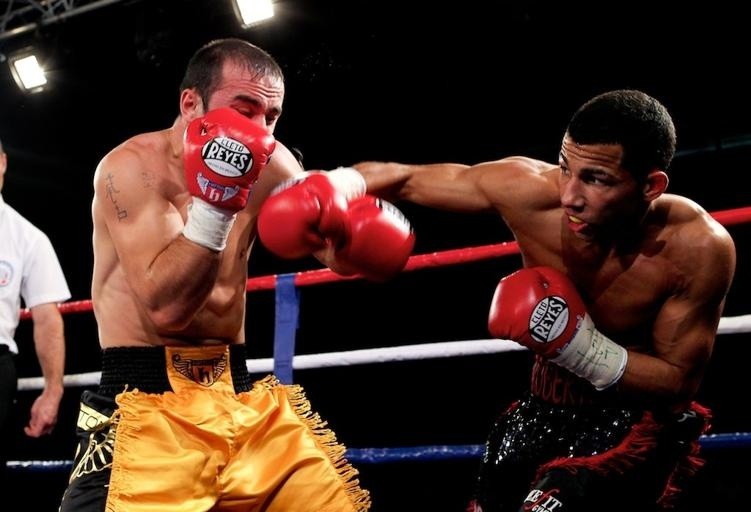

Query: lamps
left=8, top=25, right=50, bottom=94
left=232, top=1, right=277, bottom=32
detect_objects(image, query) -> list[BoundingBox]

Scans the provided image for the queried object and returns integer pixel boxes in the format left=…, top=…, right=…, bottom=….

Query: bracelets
left=182, top=195, right=237, bottom=251
left=550, top=314, right=630, bottom=393
left=327, top=164, right=368, bottom=210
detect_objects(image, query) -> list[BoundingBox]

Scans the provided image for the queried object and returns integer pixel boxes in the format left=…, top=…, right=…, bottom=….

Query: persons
left=255, top=88, right=740, bottom=512
left=0, top=140, right=74, bottom=512
left=55, top=38, right=418, bottom=512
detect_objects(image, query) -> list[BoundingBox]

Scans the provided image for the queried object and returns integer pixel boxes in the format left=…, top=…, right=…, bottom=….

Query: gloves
left=255, top=167, right=416, bottom=283
left=485, top=266, right=629, bottom=392
left=179, top=106, right=276, bottom=251
